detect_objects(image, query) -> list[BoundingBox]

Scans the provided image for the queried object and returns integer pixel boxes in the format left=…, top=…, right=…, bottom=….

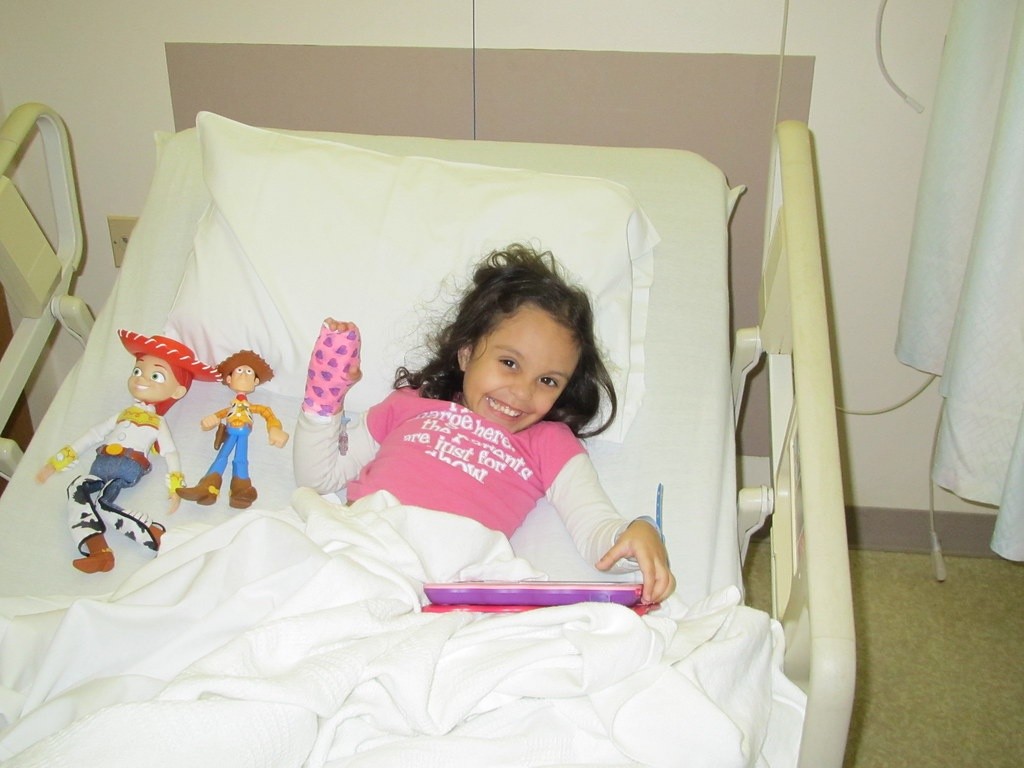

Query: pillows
left=164, top=110, right=660, bottom=445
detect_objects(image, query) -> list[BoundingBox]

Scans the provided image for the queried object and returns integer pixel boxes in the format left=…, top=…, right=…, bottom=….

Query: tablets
left=424, top=579, right=645, bottom=607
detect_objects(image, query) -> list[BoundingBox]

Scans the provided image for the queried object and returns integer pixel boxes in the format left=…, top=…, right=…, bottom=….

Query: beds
left=0, top=104, right=859, bottom=768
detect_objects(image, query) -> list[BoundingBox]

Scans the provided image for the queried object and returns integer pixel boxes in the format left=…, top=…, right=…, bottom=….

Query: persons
left=175, top=350, right=289, bottom=508
left=38, top=328, right=224, bottom=573
left=292, top=246, right=678, bottom=604
